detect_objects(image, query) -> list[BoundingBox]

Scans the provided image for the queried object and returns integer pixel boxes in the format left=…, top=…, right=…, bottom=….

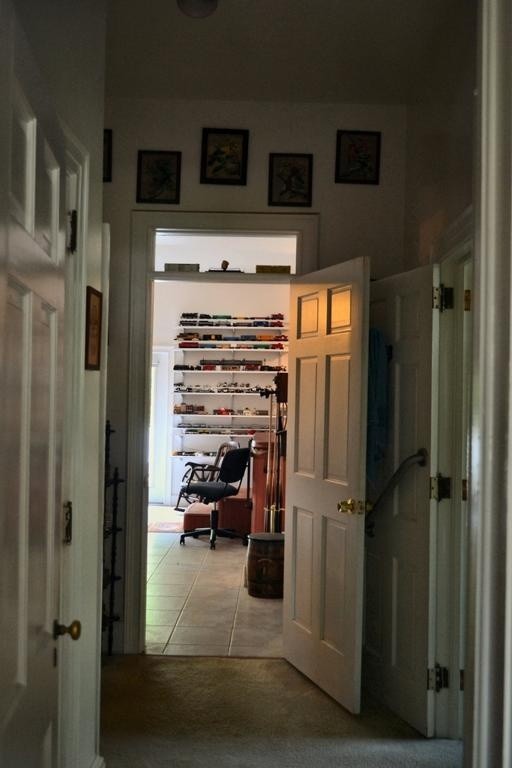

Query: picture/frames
left=266, top=151, right=314, bottom=208
left=137, top=149, right=181, bottom=204
left=334, top=126, right=382, bottom=188
left=85, top=284, right=102, bottom=371
left=103, top=127, right=114, bottom=184
left=198, top=124, right=248, bottom=186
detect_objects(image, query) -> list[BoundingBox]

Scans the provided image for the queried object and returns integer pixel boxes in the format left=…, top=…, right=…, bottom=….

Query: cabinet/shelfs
left=178, top=312, right=277, bottom=437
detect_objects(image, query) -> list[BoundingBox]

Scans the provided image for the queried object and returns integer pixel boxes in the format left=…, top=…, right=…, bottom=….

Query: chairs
left=180, top=449, right=248, bottom=549
left=174, top=441, right=241, bottom=513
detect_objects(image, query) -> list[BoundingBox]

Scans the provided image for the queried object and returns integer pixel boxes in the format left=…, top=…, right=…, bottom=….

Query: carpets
left=148, top=504, right=185, bottom=533
